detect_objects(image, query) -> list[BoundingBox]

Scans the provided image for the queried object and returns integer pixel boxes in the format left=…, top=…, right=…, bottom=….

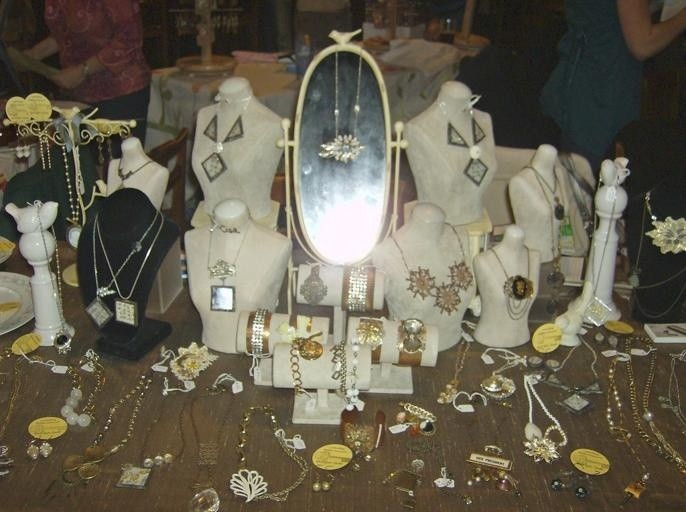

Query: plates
left=0, top=269, right=34, bottom=336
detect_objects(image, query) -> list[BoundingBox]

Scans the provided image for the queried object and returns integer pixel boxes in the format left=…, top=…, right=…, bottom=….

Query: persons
left=534, top=0, right=686, bottom=182
left=12, top=0, right=155, bottom=166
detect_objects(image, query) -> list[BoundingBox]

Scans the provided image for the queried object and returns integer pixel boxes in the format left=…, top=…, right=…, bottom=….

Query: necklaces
left=2, top=41, right=686, bottom=512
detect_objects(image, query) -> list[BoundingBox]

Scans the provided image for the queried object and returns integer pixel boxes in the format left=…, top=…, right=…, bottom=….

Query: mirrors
left=277, top=29, right=409, bottom=313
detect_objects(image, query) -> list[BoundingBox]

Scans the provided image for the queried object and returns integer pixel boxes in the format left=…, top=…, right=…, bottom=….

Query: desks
left=147, top=41, right=461, bottom=166
